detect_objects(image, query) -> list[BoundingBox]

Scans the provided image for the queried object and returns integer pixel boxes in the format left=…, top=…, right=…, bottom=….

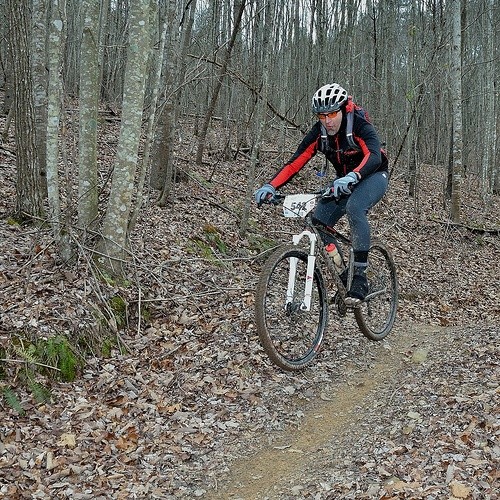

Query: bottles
left=326, top=242, right=344, bottom=273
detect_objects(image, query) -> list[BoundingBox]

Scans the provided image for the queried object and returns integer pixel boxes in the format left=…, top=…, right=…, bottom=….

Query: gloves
left=255, top=184, right=276, bottom=204
left=333, top=172, right=359, bottom=197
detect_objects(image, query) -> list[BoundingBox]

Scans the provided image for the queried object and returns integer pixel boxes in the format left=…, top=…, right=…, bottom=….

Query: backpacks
left=321, top=95, right=370, bottom=152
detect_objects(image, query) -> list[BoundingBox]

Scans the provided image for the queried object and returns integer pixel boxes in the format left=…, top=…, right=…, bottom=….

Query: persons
left=253, top=83, right=390, bottom=309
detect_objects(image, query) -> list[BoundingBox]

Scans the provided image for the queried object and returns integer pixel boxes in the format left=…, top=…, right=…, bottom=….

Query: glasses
left=317, top=109, right=341, bottom=119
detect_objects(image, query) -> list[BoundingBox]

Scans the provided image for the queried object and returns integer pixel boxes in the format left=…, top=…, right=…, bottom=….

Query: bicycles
left=255, top=182, right=399, bottom=370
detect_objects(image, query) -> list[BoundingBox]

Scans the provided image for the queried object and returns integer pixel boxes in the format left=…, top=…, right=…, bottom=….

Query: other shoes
left=331, top=269, right=348, bottom=302
left=344, top=275, right=369, bottom=305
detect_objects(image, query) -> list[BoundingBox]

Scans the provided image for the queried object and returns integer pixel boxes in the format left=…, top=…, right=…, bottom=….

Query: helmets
left=311, top=83, right=348, bottom=113
